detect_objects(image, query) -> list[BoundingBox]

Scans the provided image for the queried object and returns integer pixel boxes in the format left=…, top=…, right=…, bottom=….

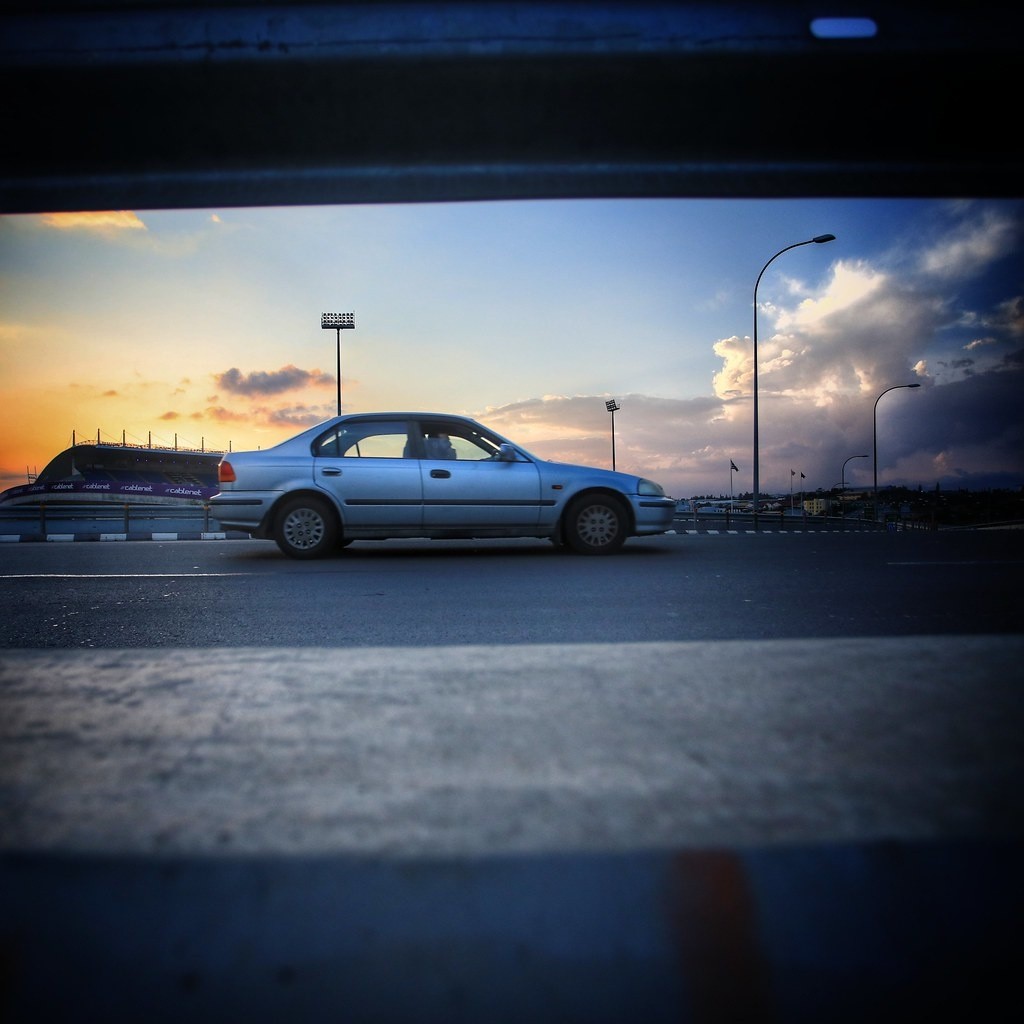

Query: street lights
left=605, top=398, right=621, bottom=472
left=320, top=310, right=357, bottom=415
left=829, top=481, right=850, bottom=516
left=873, top=383, right=921, bottom=521
left=751, top=232, right=838, bottom=513
left=842, top=454, right=869, bottom=518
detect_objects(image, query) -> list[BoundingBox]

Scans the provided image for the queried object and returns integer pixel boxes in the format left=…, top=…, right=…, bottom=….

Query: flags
left=791, top=471, right=795, bottom=476
left=801, top=473, right=805, bottom=478
left=730, top=461, right=739, bottom=472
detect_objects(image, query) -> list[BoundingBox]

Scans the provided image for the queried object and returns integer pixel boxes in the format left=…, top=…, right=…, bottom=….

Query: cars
left=205, top=411, right=679, bottom=562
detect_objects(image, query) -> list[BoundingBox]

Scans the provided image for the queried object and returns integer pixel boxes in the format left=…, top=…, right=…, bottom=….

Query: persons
left=427, top=432, right=494, bottom=460
